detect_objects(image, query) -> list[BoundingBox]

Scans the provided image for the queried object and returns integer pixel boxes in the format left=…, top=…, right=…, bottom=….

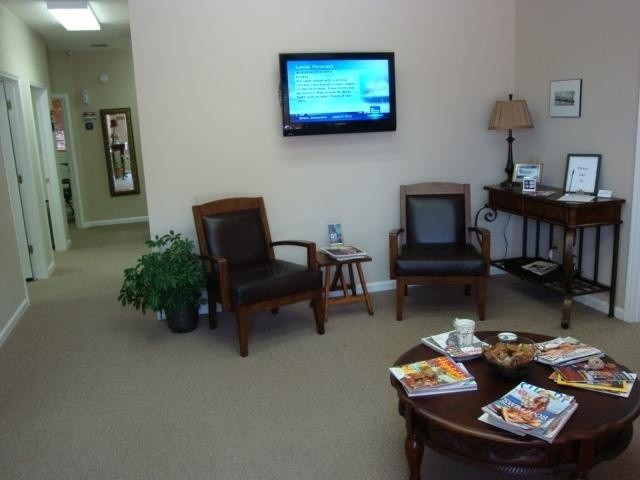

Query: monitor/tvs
left=278, top=51, right=397, bottom=137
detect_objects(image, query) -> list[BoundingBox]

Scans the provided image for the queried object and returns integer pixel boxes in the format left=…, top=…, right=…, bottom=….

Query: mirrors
left=99, top=107, right=141, bottom=197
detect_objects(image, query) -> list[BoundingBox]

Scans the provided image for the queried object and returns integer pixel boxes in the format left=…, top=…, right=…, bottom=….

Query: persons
left=501, top=391, right=560, bottom=427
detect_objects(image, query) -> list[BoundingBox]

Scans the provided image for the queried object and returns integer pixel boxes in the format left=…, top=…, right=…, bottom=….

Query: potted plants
left=115, top=155, right=126, bottom=178
left=118, top=231, right=210, bottom=333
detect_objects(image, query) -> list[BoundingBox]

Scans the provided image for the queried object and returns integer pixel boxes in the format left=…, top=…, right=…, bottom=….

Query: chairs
left=192, top=197, right=324, bottom=357
left=389, top=182, right=490, bottom=321
left=61, top=179, right=74, bottom=216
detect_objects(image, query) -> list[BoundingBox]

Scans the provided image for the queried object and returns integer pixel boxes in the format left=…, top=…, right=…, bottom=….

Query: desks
left=389, top=329, right=640, bottom=480
left=310, top=247, right=374, bottom=323
left=474, top=182, right=626, bottom=329
left=111, top=144, right=125, bottom=180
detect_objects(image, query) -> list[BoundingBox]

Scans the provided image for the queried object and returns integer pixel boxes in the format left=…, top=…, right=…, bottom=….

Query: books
left=526, top=335, right=637, bottom=400
left=388, top=355, right=479, bottom=398
left=421, top=328, right=490, bottom=362
left=321, top=250, right=365, bottom=261
left=476, top=381, right=581, bottom=444
left=321, top=245, right=367, bottom=257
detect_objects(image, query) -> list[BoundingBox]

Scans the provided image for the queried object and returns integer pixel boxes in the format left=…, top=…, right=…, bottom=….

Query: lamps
left=111, top=120, right=119, bottom=143
left=46, top=0, right=101, bottom=32
left=488, top=93, right=534, bottom=188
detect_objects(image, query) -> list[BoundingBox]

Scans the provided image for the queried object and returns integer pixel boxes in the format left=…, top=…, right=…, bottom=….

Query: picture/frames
left=563, top=153, right=602, bottom=197
left=548, top=79, right=582, bottom=118
left=522, top=176, right=537, bottom=193
left=511, top=163, right=543, bottom=184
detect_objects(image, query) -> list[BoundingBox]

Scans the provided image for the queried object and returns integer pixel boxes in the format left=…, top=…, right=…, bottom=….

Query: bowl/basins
left=479, top=335, right=539, bottom=376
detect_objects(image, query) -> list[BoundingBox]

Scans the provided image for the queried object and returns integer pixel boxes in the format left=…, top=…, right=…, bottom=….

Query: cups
left=452, top=317, right=475, bottom=347
